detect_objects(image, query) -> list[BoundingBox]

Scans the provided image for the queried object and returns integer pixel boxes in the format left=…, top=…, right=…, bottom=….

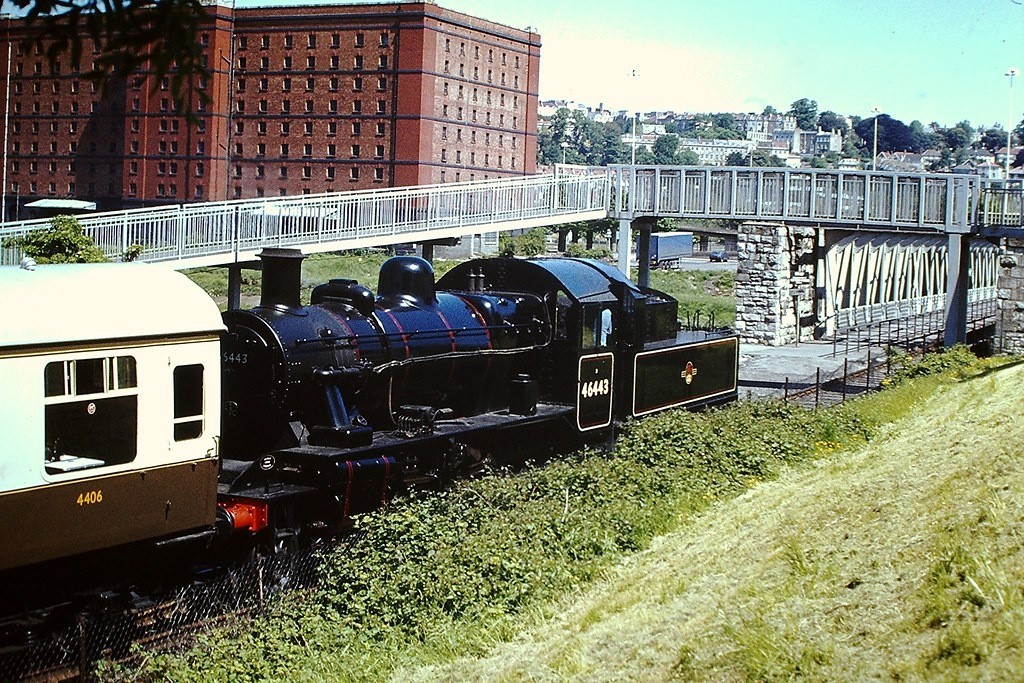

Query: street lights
left=871, top=107, right=879, bottom=222
left=628, top=68, right=640, bottom=215
left=1002, top=67, right=1018, bottom=213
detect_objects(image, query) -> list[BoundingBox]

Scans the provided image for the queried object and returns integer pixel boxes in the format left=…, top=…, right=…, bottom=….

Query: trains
left=0, top=247, right=686, bottom=683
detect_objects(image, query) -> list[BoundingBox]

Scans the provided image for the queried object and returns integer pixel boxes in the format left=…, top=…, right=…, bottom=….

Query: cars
left=709, top=250, right=729, bottom=262
